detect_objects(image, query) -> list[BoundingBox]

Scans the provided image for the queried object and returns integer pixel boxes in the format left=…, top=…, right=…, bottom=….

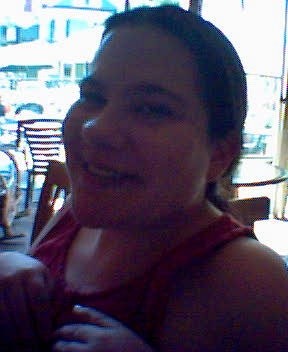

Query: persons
left=0, top=5, right=287, bottom=352
left=0, top=174, right=24, bottom=240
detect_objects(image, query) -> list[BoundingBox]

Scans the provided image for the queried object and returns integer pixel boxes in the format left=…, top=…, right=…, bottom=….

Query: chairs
left=227, top=195, right=269, bottom=235
left=30, top=159, right=71, bottom=243
left=14, top=119, right=65, bottom=214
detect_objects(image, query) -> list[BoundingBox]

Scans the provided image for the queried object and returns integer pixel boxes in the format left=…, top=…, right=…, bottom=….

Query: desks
left=227, top=156, right=288, bottom=198
